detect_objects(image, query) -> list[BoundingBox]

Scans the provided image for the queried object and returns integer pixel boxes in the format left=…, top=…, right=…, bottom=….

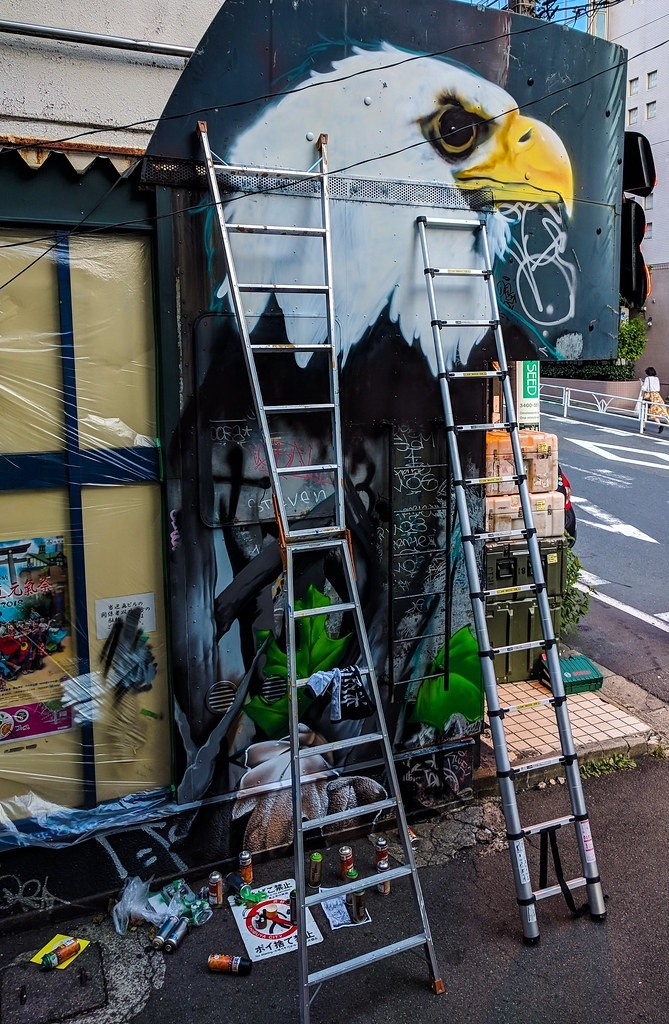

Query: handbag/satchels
left=643, top=397, right=652, bottom=408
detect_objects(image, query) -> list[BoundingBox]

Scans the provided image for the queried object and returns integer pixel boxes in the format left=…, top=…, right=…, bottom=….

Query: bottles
left=375, top=837, right=388, bottom=870
left=377, top=859, right=390, bottom=896
left=152, top=916, right=190, bottom=952
left=290, top=889, right=297, bottom=922
left=308, top=852, right=322, bottom=889
left=346, top=869, right=367, bottom=924
left=209, top=850, right=253, bottom=909
left=339, top=846, right=354, bottom=880
left=207, top=953, right=252, bottom=976
left=407, top=825, right=420, bottom=851
left=42, top=937, right=80, bottom=968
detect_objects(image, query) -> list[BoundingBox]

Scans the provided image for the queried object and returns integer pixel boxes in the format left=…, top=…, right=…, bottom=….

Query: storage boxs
left=480, top=429, right=604, bottom=694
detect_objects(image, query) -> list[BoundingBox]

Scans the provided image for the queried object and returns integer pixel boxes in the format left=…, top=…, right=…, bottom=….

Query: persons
left=638, top=367, right=667, bottom=434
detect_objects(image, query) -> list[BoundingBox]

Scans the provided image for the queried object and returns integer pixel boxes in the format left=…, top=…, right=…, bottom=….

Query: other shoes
left=639, top=428, right=646, bottom=431
left=659, top=422, right=665, bottom=433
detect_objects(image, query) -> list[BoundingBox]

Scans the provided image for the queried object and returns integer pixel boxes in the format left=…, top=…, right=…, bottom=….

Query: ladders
left=193, top=116, right=455, bottom=1023
left=413, top=213, right=608, bottom=946
